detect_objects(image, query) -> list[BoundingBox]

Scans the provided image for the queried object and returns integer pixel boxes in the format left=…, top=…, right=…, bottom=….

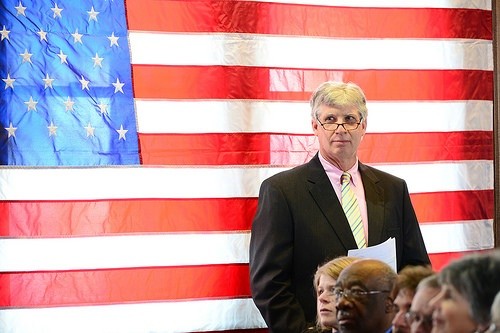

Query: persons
left=389, top=265, right=437, bottom=333
left=250, top=81, right=432, bottom=333
left=313, top=257, right=364, bottom=333
left=408, top=274, right=442, bottom=333
left=334, top=259, right=398, bottom=333
left=429, top=254, right=500, bottom=333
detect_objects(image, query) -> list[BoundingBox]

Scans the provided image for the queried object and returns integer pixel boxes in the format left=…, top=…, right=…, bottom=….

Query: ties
left=341, top=171, right=367, bottom=249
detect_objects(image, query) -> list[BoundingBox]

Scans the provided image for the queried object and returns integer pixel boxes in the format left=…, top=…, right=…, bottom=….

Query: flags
left=0, top=0, right=495, bottom=333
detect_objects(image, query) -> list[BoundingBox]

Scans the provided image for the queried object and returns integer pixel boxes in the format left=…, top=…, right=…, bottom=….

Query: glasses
left=333, top=289, right=391, bottom=299
left=406, top=312, right=433, bottom=329
left=316, top=116, right=363, bottom=131
